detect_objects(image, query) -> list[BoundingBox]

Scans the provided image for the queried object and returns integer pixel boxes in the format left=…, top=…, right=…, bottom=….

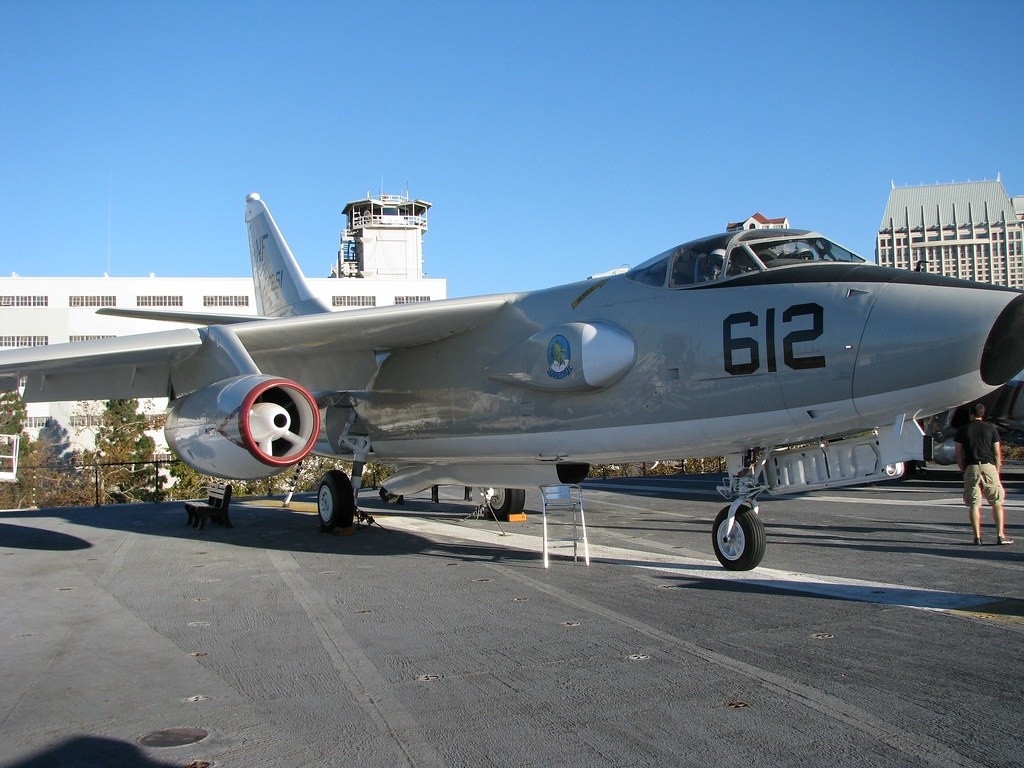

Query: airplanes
left=2, top=191, right=1024, bottom=572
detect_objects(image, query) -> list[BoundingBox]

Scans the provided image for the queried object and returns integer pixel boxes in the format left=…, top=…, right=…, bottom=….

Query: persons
left=953, top=403, right=1015, bottom=546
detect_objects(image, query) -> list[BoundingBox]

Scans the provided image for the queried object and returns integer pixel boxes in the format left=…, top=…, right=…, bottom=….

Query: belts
left=968, top=461, right=987, bottom=464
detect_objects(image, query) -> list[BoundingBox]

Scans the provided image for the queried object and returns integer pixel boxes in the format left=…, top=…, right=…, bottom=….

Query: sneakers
left=974, top=538, right=982, bottom=545
left=997, top=537, right=1014, bottom=544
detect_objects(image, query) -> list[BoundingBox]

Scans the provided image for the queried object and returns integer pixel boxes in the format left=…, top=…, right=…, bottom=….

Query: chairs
left=539, top=483, right=589, bottom=569
left=694, top=253, right=709, bottom=283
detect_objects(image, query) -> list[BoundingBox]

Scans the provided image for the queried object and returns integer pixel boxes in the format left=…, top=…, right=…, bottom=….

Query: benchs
left=184, top=482, right=233, bottom=530
